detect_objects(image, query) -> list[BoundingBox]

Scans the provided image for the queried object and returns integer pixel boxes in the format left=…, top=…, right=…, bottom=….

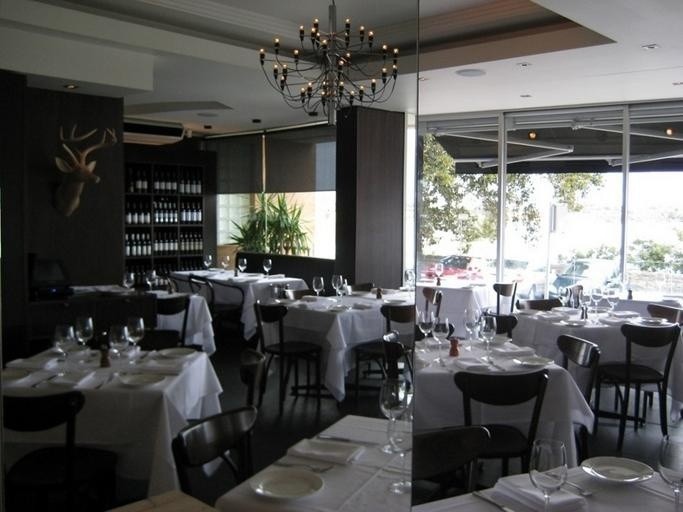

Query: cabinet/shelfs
left=122, top=144, right=215, bottom=289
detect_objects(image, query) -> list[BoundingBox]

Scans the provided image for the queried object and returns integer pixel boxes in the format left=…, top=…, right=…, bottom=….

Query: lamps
left=257, top=1, right=403, bottom=128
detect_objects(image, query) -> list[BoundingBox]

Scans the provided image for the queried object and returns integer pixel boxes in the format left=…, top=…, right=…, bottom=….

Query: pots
left=269, top=281, right=296, bottom=300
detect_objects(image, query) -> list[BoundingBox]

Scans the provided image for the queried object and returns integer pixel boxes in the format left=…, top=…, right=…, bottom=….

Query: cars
left=422, top=255, right=636, bottom=301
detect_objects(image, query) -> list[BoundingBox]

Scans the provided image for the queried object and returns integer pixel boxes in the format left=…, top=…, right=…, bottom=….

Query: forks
left=272, top=457, right=333, bottom=473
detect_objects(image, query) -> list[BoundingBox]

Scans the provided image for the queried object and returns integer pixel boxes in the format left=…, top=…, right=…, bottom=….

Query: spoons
left=546, top=469, right=593, bottom=497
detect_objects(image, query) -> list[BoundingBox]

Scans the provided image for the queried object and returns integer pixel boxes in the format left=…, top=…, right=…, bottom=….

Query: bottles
left=436, top=278, right=442, bottom=285
left=377, top=286, right=382, bottom=299
left=166, top=281, right=175, bottom=294
left=126, top=167, right=202, bottom=286
left=383, top=328, right=400, bottom=342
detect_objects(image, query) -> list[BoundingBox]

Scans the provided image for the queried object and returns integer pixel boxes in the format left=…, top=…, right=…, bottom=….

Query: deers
left=54, top=120, right=118, bottom=217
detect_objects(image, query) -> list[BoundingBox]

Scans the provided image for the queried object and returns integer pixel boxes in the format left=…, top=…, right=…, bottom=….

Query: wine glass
left=555, top=282, right=621, bottom=322
left=528, top=437, right=568, bottom=510
left=118, top=269, right=157, bottom=294
left=376, top=378, right=411, bottom=498
left=405, top=271, right=414, bottom=296
left=417, top=306, right=500, bottom=362
left=51, top=314, right=144, bottom=380
left=434, top=261, right=444, bottom=278
left=203, top=254, right=272, bottom=278
left=655, top=435, right=683, bottom=510
left=312, top=274, right=347, bottom=304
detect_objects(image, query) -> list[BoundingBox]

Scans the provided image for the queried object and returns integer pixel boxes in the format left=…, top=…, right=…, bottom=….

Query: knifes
left=93, top=372, right=117, bottom=389
left=313, top=431, right=380, bottom=447
left=31, top=371, right=58, bottom=390
left=140, top=350, right=156, bottom=360
left=487, top=357, right=507, bottom=372
left=473, top=492, right=515, bottom=512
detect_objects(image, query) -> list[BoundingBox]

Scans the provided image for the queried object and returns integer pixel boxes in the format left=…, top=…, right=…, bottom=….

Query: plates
left=159, top=347, right=197, bottom=358
left=581, top=454, right=653, bottom=484
left=514, top=356, right=554, bottom=367
left=274, top=299, right=298, bottom=304
left=122, top=372, right=165, bottom=388
left=383, top=298, right=408, bottom=304
left=0, top=366, right=31, bottom=385
left=351, top=290, right=369, bottom=296
left=493, top=336, right=510, bottom=343
left=642, top=317, right=667, bottom=324
left=562, top=320, right=586, bottom=326
left=249, top=472, right=324, bottom=498
left=327, top=306, right=351, bottom=313
